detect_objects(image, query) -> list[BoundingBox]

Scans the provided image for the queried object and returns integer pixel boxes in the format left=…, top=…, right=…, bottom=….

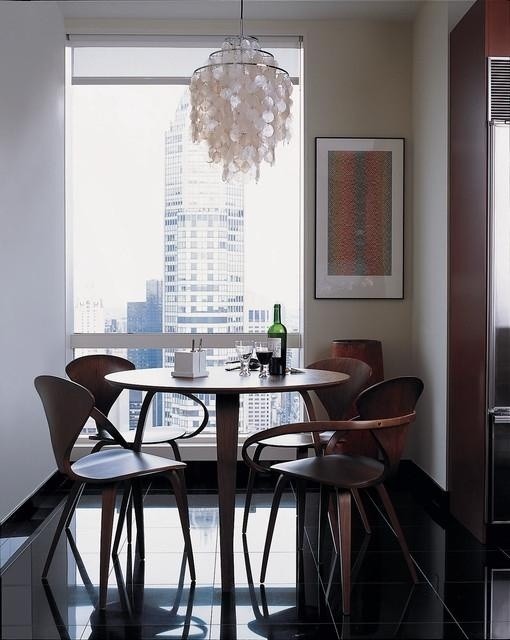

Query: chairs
left=65, top=355, right=209, bottom=543
left=242, top=357, right=373, bottom=553
left=261, top=377, right=424, bottom=615
left=33, top=375, right=196, bottom=608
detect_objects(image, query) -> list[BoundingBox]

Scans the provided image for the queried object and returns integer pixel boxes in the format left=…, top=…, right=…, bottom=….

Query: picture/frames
left=315, top=136, right=406, bottom=301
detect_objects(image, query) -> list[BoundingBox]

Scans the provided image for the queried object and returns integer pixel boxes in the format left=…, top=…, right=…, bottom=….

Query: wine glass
left=234, top=342, right=254, bottom=377
left=254, top=341, right=273, bottom=378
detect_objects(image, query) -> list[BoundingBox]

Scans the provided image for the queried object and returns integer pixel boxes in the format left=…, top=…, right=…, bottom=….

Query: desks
left=100, top=365, right=347, bottom=597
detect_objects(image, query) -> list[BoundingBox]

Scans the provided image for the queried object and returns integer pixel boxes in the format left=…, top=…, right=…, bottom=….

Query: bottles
left=267, top=303, right=288, bottom=376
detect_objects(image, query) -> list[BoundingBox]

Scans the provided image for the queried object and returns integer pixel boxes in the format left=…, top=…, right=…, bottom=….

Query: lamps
left=183, top=0, right=296, bottom=183
